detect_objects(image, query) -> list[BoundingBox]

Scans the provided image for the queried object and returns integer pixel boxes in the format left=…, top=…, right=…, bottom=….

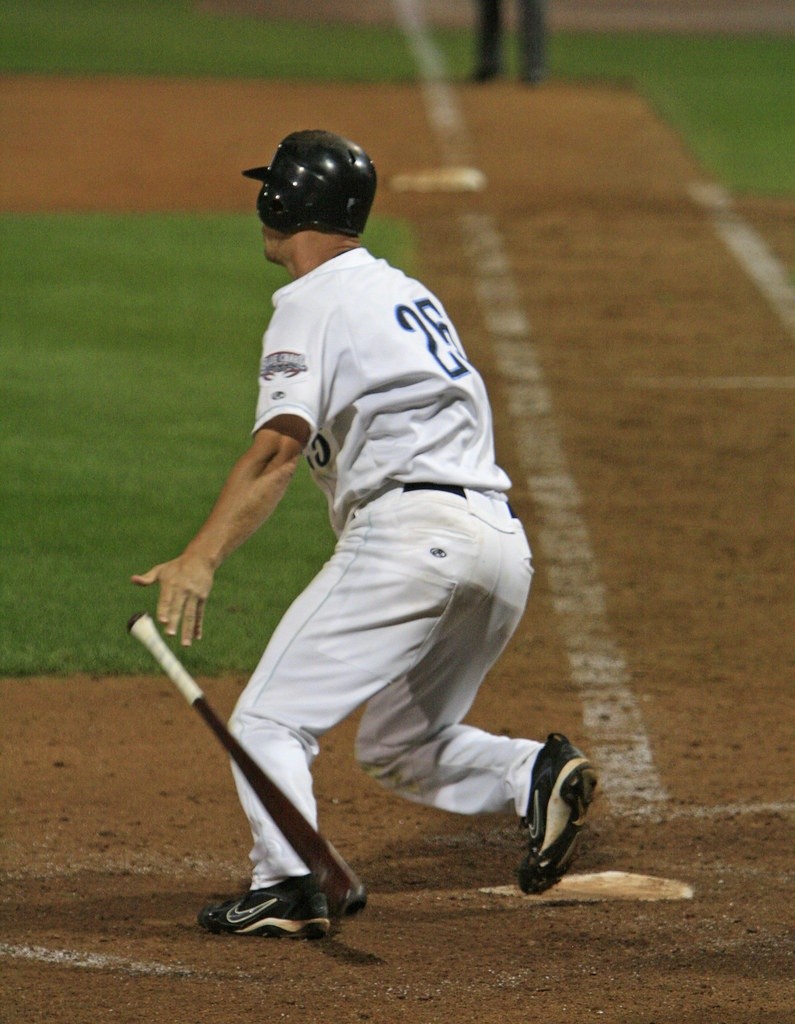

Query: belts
left=403, top=482, right=519, bottom=518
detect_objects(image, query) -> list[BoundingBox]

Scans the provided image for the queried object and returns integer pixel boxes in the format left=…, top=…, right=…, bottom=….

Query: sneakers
left=197, top=871, right=331, bottom=941
left=518, top=732, right=597, bottom=895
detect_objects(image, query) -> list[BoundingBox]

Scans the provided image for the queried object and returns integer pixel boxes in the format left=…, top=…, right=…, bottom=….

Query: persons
left=130, top=132, right=596, bottom=939
left=473, top=0, right=544, bottom=84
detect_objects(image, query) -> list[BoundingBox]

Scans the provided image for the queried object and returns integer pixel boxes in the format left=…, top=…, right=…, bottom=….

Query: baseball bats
left=129, top=609, right=366, bottom=917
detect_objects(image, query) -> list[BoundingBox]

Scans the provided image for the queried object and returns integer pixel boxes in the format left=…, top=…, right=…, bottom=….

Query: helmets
left=241, top=129, right=378, bottom=234
